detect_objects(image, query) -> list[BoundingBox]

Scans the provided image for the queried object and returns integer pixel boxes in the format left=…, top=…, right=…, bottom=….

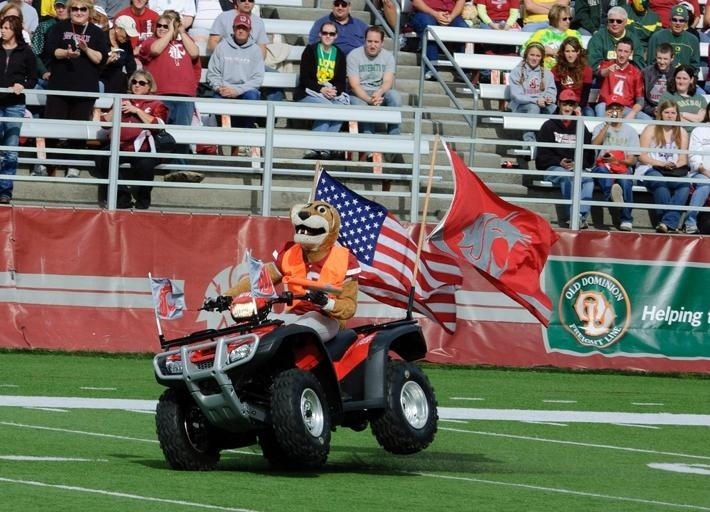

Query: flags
left=151, top=278, right=187, bottom=319
left=425, top=138, right=561, bottom=327
left=248, top=257, right=280, bottom=300
left=308, top=167, right=464, bottom=335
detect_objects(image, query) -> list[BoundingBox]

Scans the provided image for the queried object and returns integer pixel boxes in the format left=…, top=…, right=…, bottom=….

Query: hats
left=114, top=14, right=141, bottom=38
left=230, top=12, right=252, bottom=30
left=671, top=2, right=696, bottom=18
left=54, top=0, right=68, bottom=10
left=604, top=92, right=626, bottom=108
left=558, top=87, right=579, bottom=104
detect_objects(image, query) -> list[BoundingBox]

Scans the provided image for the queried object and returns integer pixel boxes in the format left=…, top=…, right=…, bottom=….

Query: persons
left=2, top=1, right=710, bottom=235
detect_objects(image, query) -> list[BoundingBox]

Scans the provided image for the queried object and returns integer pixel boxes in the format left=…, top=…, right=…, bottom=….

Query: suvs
left=145, top=285, right=443, bottom=472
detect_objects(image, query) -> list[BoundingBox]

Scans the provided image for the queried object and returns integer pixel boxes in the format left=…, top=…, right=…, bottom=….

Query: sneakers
left=28, top=164, right=85, bottom=181
left=424, top=70, right=438, bottom=80
left=0, top=190, right=15, bottom=205
left=580, top=217, right=589, bottom=230
left=616, top=218, right=635, bottom=231
left=654, top=219, right=701, bottom=234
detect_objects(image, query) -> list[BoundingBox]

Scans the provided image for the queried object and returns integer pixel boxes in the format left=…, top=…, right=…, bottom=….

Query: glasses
left=332, top=1, right=348, bottom=8
left=559, top=15, right=574, bottom=23
left=130, top=77, right=151, bottom=88
left=69, top=4, right=89, bottom=13
left=320, top=29, right=338, bottom=37
left=669, top=16, right=689, bottom=24
left=605, top=17, right=627, bottom=25
left=155, top=21, right=173, bottom=30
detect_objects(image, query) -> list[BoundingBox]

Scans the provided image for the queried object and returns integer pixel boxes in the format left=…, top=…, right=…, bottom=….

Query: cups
left=611, top=110, right=619, bottom=118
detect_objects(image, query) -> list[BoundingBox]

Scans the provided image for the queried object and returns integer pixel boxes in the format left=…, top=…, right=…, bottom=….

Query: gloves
left=214, top=293, right=233, bottom=312
left=308, top=290, right=329, bottom=307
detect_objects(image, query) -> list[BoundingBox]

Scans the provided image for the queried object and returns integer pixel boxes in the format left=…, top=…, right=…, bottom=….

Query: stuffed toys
left=205, top=202, right=360, bottom=345
